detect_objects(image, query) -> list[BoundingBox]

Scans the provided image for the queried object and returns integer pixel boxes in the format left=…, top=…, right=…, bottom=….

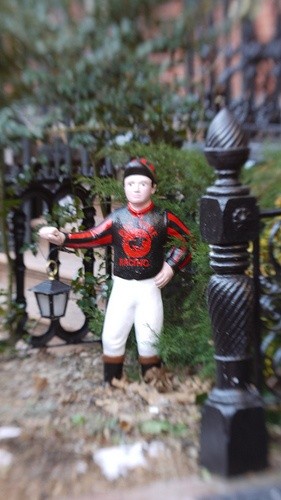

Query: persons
left=37, top=158, right=196, bottom=390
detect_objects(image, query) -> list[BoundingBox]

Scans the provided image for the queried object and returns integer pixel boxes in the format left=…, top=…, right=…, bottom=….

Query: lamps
left=27, top=277, right=72, bottom=320
